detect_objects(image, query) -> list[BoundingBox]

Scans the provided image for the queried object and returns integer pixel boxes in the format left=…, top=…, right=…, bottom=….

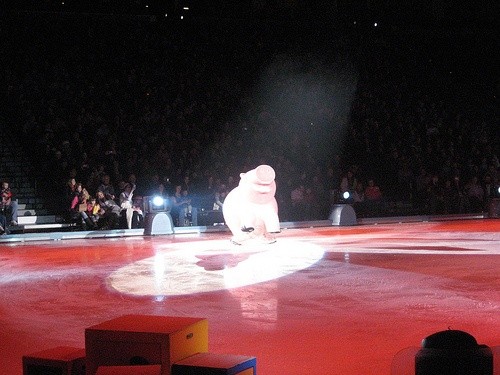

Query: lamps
left=144, top=195, right=174, bottom=235
left=328, top=190, right=357, bottom=226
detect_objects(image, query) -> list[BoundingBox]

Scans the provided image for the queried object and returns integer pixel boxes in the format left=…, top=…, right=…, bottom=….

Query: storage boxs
left=22, top=315, right=256, bottom=375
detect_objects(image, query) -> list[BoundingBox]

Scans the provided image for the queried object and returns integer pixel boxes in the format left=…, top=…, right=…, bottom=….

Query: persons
left=0, top=33, right=500, bottom=238
